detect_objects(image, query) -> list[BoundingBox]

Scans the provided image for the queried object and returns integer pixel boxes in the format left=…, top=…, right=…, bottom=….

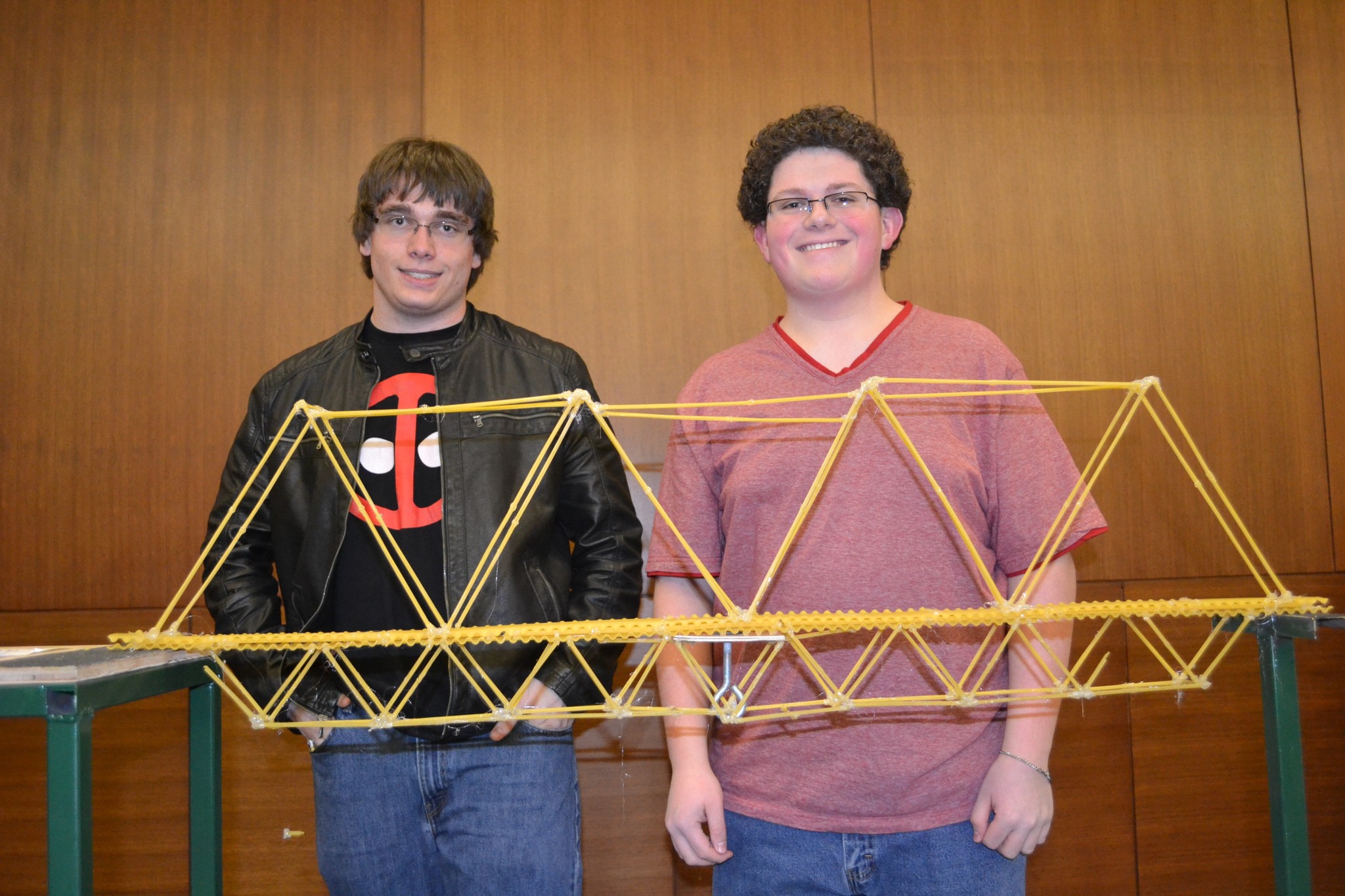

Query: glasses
left=370, top=215, right=476, bottom=247
left=763, top=191, right=883, bottom=217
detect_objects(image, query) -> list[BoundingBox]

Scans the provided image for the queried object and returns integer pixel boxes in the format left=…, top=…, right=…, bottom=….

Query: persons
left=646, top=104, right=1110, bottom=896
left=199, top=136, right=643, bottom=896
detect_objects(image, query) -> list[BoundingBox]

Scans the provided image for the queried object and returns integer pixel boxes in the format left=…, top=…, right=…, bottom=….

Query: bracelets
left=999, top=750, right=1051, bottom=781
